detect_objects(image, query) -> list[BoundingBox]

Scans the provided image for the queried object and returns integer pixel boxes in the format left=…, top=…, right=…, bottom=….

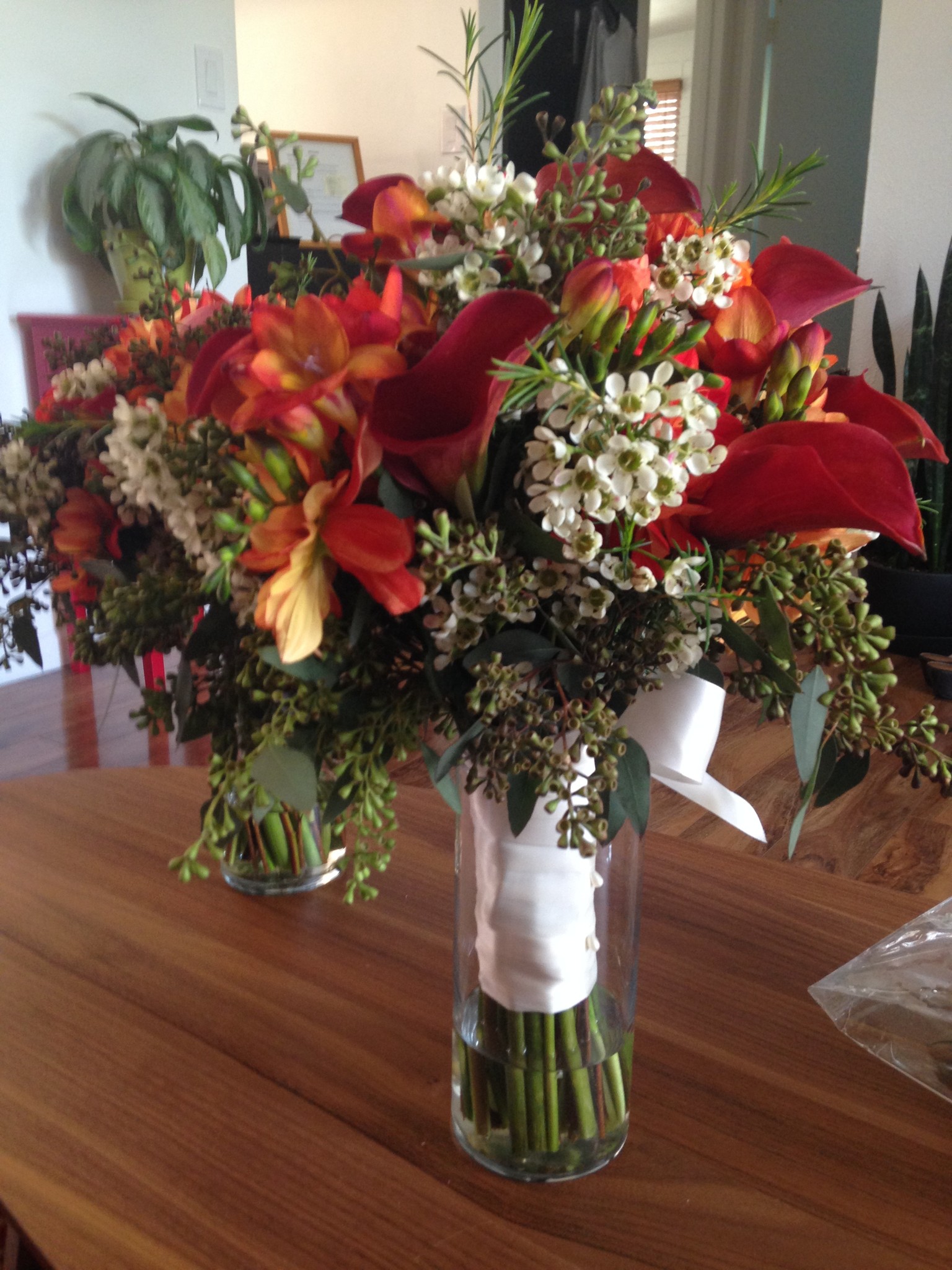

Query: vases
left=450, top=741, right=640, bottom=1185
left=215, top=789, right=345, bottom=895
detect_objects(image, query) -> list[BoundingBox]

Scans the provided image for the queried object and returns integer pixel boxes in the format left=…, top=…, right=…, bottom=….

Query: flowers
left=0, top=0, right=952, bottom=1173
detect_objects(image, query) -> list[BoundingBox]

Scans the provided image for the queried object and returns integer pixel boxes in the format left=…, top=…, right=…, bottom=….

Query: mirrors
left=266, top=131, right=372, bottom=249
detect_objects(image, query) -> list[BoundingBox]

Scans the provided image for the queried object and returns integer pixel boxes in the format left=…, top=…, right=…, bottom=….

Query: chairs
left=19, top=313, right=211, bottom=717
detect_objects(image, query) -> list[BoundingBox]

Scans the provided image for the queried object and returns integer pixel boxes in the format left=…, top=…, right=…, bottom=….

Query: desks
left=0, top=767, right=952, bottom=1270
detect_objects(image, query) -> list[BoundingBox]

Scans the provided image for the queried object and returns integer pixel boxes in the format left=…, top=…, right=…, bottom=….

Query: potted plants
left=60, top=91, right=268, bottom=302
left=858, top=237, right=951, bottom=657
